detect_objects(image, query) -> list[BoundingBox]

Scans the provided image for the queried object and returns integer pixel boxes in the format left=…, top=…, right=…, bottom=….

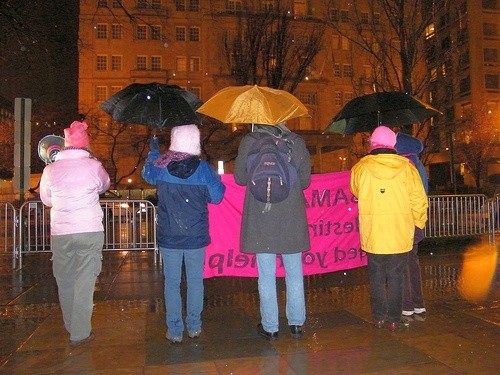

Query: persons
left=39, top=120, right=111, bottom=345
left=232, top=121, right=313, bottom=340
left=392, top=131, right=428, bottom=315
left=351, top=125, right=428, bottom=330
left=141, top=123, right=227, bottom=344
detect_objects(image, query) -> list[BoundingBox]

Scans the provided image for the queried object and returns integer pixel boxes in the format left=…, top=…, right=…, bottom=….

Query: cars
left=99, top=191, right=139, bottom=219
left=485, top=194, right=500, bottom=218
left=136, top=196, right=159, bottom=225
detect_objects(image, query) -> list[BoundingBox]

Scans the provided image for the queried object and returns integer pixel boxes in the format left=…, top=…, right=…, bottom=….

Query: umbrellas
left=322, top=89, right=443, bottom=137
left=100, top=80, right=202, bottom=137
left=194, top=84, right=309, bottom=132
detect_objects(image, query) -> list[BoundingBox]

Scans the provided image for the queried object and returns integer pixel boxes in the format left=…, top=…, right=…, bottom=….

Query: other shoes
left=390, top=322, right=401, bottom=331
left=70, top=333, right=95, bottom=347
left=291, top=324, right=303, bottom=336
left=402, top=310, right=414, bottom=316
left=257, top=323, right=278, bottom=340
left=166, top=328, right=183, bottom=343
left=375, top=320, right=385, bottom=326
left=190, top=327, right=202, bottom=339
left=414, top=307, right=426, bottom=314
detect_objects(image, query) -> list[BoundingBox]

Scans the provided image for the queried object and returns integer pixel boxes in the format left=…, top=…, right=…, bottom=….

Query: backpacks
left=247, top=129, right=301, bottom=203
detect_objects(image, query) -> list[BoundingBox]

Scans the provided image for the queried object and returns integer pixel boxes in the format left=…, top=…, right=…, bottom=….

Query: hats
left=169, top=124, right=202, bottom=154
left=63, top=121, right=90, bottom=148
left=369, top=126, right=397, bottom=146
